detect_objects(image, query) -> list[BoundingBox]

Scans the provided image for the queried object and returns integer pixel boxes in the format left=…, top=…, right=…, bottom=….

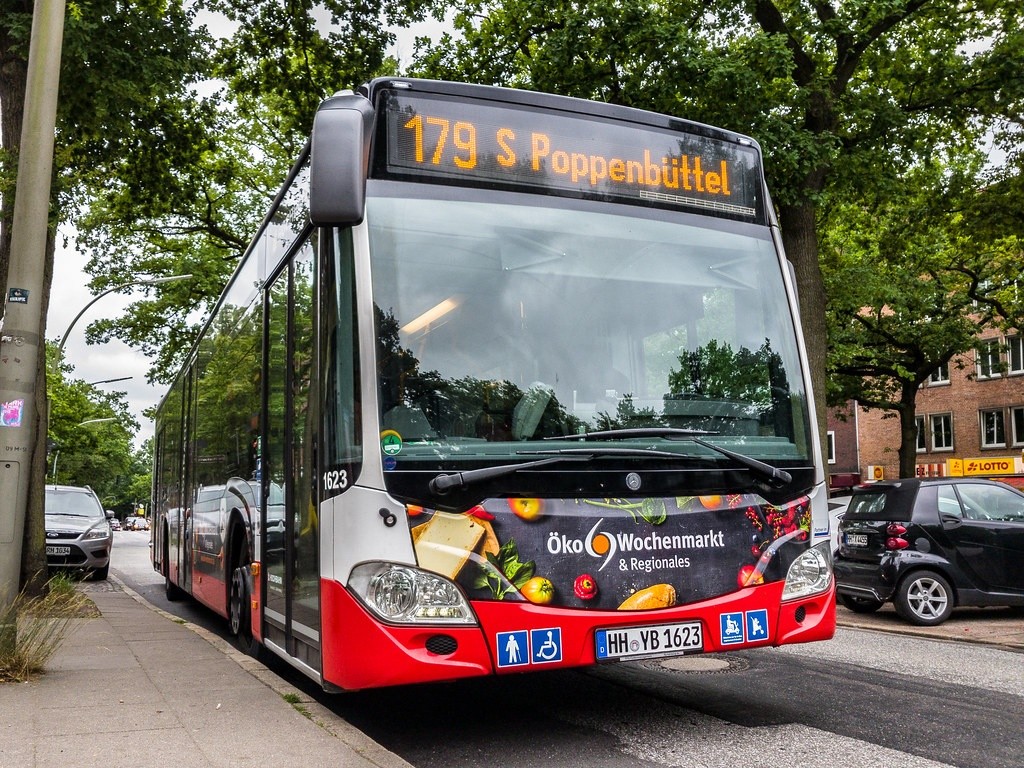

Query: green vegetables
left=583, top=496, right=697, bottom=525
left=461, top=536, right=537, bottom=600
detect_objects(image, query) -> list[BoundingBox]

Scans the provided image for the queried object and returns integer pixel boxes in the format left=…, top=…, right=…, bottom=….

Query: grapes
left=725, top=494, right=784, bottom=540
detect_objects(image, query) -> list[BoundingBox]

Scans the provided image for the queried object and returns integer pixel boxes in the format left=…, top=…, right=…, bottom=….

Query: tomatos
left=521, top=577, right=554, bottom=604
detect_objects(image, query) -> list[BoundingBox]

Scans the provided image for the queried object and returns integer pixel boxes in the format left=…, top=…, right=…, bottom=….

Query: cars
left=131, top=518, right=148, bottom=530
left=123, top=517, right=136, bottom=530
left=166, top=507, right=185, bottom=530
left=830, top=478, right=1024, bottom=624
left=110, top=519, right=122, bottom=531
left=826, top=492, right=973, bottom=545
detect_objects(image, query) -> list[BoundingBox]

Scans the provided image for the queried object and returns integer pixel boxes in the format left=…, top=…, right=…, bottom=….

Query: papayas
left=617, top=584, right=676, bottom=610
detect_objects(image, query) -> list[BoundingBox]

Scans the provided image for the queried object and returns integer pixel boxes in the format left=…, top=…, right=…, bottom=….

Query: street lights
left=46, top=273, right=195, bottom=428
left=52, top=418, right=117, bottom=485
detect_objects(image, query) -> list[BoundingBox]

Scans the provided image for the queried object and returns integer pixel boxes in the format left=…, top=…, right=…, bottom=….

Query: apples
left=507, top=498, right=545, bottom=522
left=737, top=566, right=764, bottom=589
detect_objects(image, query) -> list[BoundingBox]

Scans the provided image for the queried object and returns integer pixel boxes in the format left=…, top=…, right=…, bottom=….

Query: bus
left=148, top=72, right=837, bottom=691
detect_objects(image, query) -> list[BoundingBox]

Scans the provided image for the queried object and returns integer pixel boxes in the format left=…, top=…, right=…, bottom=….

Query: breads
left=413, top=509, right=485, bottom=582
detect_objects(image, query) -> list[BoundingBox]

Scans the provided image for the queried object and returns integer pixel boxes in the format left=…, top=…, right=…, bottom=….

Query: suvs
left=186, top=483, right=226, bottom=563
left=44, top=485, right=115, bottom=580
left=227, top=478, right=300, bottom=556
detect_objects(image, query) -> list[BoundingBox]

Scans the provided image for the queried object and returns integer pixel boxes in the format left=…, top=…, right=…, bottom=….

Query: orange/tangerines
left=699, top=495, right=723, bottom=510
